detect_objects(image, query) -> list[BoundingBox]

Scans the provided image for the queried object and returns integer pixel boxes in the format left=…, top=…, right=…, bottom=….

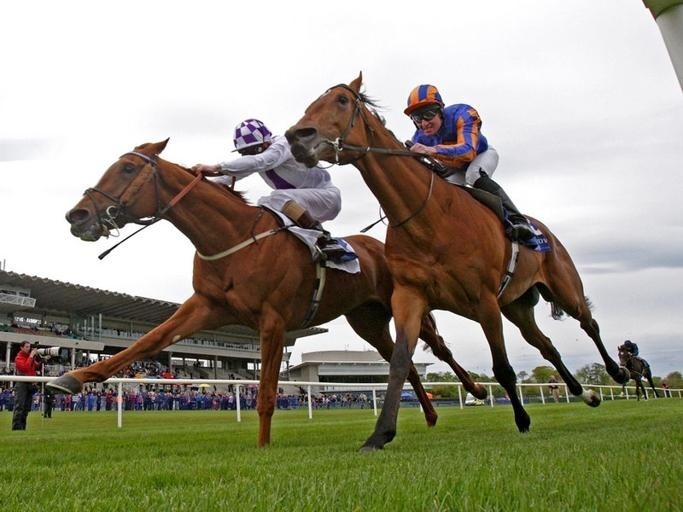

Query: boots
left=473, top=168, right=534, bottom=240
left=282, top=201, right=345, bottom=258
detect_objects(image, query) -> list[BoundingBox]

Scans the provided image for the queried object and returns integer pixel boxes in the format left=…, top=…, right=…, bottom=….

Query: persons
left=187, top=118, right=358, bottom=264
left=8, top=340, right=32, bottom=431
left=546, top=374, right=562, bottom=403
left=621, top=340, right=639, bottom=357
left=397, top=82, right=549, bottom=253
left=0, top=360, right=376, bottom=412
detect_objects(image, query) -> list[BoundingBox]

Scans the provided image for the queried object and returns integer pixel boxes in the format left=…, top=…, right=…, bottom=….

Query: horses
left=44, top=136, right=488, bottom=450
left=283, top=69, right=632, bottom=453
left=617, top=345, right=661, bottom=400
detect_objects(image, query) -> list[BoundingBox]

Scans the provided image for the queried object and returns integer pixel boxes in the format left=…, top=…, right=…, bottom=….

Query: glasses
left=410, top=105, right=441, bottom=123
left=239, top=144, right=263, bottom=155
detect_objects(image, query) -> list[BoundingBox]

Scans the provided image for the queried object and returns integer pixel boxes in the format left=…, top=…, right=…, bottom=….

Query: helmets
left=232, top=119, right=272, bottom=152
left=403, top=84, right=444, bottom=116
